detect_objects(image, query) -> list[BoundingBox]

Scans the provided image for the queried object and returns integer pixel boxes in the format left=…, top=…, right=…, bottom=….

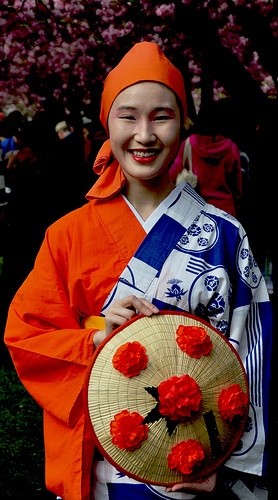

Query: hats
left=99, top=42, right=188, bottom=134
left=84, top=310, right=249, bottom=487
left=55, top=121, right=67, bottom=132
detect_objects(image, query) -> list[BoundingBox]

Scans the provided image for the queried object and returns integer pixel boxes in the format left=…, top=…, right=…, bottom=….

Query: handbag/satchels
left=176, top=138, right=197, bottom=189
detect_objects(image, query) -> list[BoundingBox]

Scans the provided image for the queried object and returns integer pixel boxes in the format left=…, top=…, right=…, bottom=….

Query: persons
left=167, top=104, right=242, bottom=218
left=0, top=91, right=111, bottom=233
left=4, top=42, right=271, bottom=500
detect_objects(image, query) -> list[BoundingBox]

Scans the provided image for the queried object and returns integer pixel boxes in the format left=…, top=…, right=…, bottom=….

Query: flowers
left=168, top=440, right=205, bottom=474
left=218, top=384, right=248, bottom=423
left=110, top=410, right=148, bottom=451
left=158, top=374, right=203, bottom=421
left=112, top=341, right=148, bottom=378
left=176, top=325, right=213, bottom=359
